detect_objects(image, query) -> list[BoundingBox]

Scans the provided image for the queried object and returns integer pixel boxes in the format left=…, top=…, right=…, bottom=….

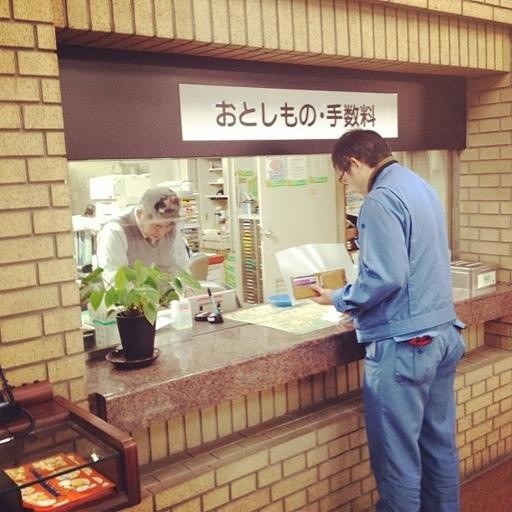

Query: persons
left=96, top=186, right=191, bottom=292
left=311, top=130, right=467, bottom=512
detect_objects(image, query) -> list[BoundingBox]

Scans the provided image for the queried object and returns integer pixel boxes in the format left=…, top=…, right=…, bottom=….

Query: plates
left=266, top=293, right=293, bottom=306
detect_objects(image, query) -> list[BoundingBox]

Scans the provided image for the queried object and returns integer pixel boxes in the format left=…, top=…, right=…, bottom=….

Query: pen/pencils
left=208, top=288, right=219, bottom=315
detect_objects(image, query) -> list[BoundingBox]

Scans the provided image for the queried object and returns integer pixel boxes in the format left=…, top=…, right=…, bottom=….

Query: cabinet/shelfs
left=196, top=153, right=345, bottom=308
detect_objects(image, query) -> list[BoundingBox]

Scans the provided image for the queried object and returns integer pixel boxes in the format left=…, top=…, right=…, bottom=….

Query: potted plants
left=78, top=259, right=203, bottom=360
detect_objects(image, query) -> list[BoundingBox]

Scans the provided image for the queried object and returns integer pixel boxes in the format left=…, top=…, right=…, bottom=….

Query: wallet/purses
left=290, top=268, right=347, bottom=300
